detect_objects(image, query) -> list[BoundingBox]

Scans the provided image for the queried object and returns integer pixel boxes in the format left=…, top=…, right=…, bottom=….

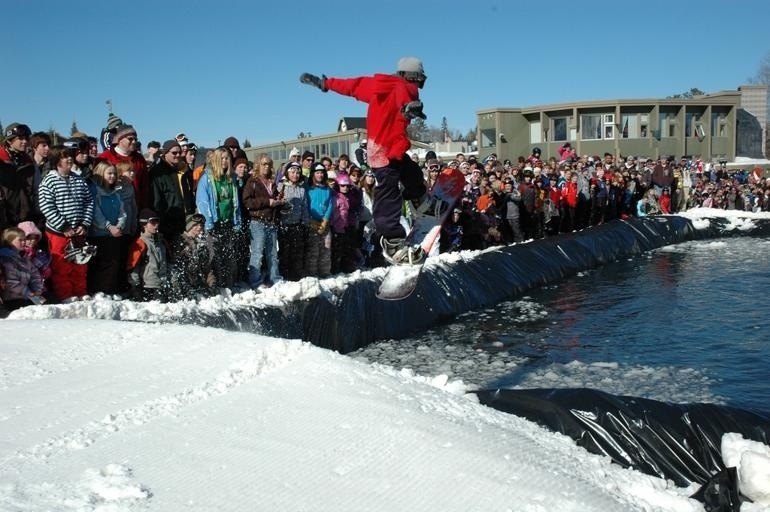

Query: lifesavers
left=636, top=199, right=658, bottom=215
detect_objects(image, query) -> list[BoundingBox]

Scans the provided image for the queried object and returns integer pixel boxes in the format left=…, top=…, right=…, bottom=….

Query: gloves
left=401, top=99, right=426, bottom=119
left=299, top=73, right=328, bottom=93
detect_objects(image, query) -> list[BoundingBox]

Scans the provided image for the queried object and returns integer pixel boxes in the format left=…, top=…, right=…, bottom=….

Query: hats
left=286, top=147, right=362, bottom=186
left=18, top=221, right=42, bottom=243
left=185, top=214, right=205, bottom=231
left=224, top=137, right=239, bottom=147
left=64, top=112, right=197, bottom=156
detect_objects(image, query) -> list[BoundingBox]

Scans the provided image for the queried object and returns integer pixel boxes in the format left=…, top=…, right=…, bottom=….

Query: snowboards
left=375, top=167, right=466, bottom=301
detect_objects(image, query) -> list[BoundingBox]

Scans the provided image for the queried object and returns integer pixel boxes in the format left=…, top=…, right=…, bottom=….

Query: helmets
left=63, top=236, right=95, bottom=266
left=2, top=123, right=32, bottom=142
left=399, top=57, right=425, bottom=74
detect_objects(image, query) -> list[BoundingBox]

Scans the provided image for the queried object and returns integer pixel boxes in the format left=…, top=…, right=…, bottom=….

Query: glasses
left=12, top=124, right=31, bottom=137
left=148, top=216, right=161, bottom=226
left=192, top=213, right=206, bottom=222
left=428, top=164, right=441, bottom=172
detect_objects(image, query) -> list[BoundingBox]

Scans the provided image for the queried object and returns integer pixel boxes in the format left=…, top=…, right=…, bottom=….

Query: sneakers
left=415, top=192, right=449, bottom=215
left=384, top=239, right=416, bottom=259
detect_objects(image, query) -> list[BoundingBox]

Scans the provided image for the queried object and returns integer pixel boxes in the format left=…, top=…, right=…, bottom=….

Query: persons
left=0, top=123, right=769, bottom=312
left=299, top=54, right=449, bottom=267
